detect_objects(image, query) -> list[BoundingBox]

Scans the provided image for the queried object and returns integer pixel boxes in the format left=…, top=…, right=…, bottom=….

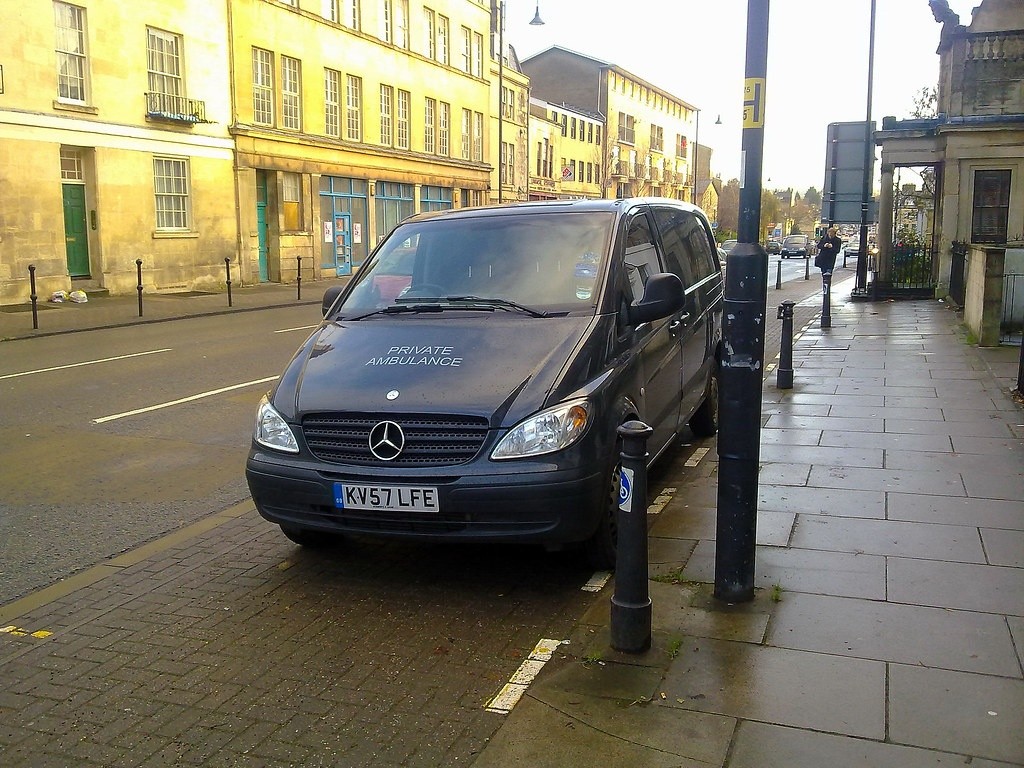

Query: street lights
left=694, top=109, right=724, bottom=206
left=497, top=1, right=545, bottom=203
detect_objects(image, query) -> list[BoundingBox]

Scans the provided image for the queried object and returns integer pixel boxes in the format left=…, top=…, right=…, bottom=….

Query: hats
left=827, top=227, right=836, bottom=232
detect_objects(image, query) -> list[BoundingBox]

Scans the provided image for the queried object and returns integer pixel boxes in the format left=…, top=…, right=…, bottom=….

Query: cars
left=836, top=224, right=860, bottom=257
left=768, top=234, right=820, bottom=259
left=247, top=196, right=737, bottom=554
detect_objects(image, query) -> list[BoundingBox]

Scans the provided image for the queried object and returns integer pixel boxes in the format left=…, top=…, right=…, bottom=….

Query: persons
left=817, top=227, right=841, bottom=275
